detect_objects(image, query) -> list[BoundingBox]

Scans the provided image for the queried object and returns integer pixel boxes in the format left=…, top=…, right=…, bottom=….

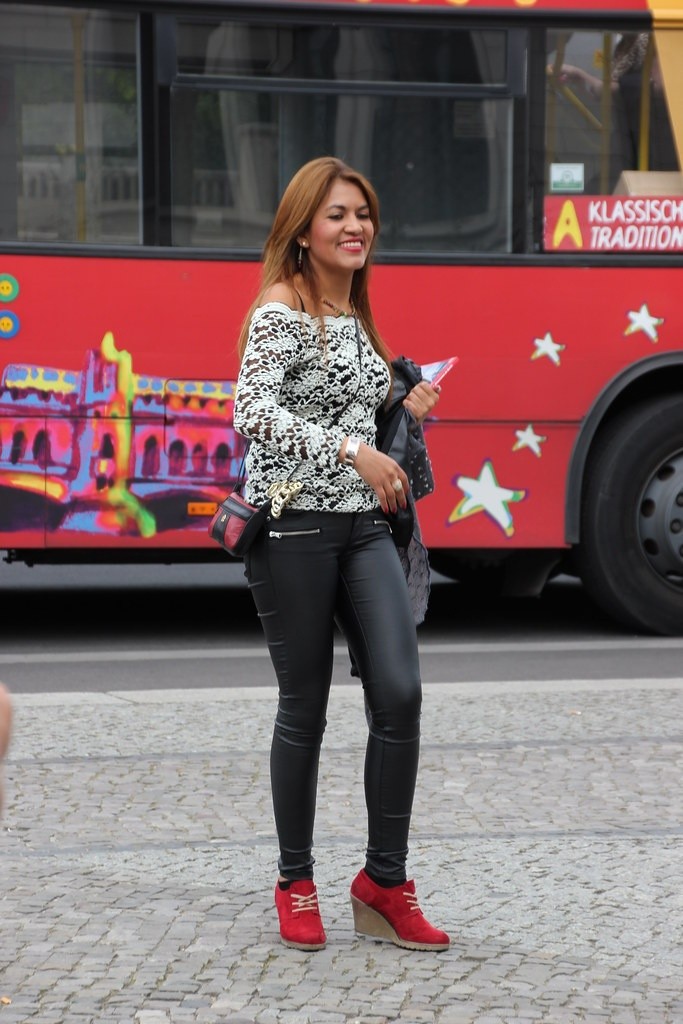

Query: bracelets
left=342, top=437, right=360, bottom=466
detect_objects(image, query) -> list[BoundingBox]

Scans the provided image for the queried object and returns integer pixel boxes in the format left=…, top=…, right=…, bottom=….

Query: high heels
left=273, top=880, right=327, bottom=951
left=347, top=868, right=451, bottom=952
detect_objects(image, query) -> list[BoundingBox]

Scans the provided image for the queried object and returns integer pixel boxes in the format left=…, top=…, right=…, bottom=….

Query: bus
left=1, top=0, right=683, bottom=638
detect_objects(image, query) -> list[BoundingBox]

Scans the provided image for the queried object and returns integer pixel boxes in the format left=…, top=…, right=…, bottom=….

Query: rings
left=391, top=478, right=402, bottom=491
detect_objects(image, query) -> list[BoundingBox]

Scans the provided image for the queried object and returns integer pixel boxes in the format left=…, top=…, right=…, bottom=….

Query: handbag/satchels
left=208, top=490, right=277, bottom=557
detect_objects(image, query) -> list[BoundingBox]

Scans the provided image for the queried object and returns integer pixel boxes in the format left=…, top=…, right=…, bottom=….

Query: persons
left=233, top=157, right=453, bottom=952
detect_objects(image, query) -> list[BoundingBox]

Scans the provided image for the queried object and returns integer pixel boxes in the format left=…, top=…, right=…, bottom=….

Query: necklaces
left=323, top=297, right=356, bottom=317
